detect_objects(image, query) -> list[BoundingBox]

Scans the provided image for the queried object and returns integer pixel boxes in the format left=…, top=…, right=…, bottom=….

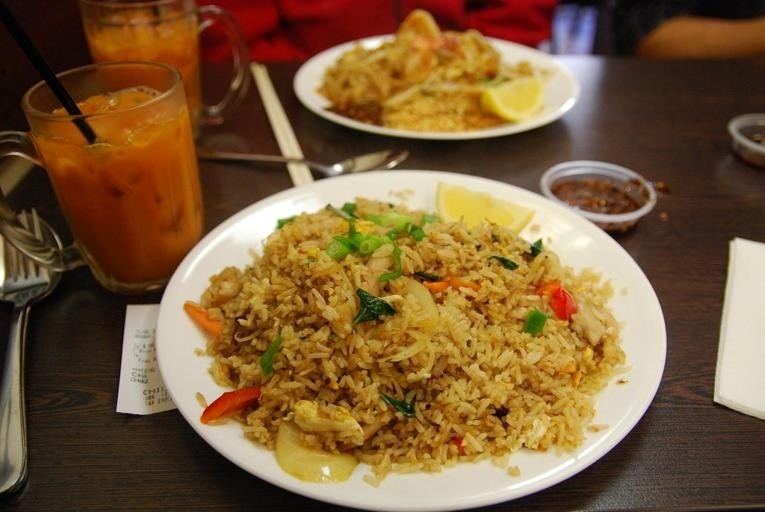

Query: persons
left=592, top=0, right=764, bottom=61
left=189, top=3, right=563, bottom=67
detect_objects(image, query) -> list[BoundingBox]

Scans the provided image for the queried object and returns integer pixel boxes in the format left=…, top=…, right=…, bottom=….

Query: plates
left=154, top=169, right=668, bottom=512
left=292, top=31, right=580, bottom=143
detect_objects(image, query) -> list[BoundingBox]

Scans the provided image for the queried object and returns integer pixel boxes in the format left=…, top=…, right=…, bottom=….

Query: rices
left=194, top=196, right=628, bottom=488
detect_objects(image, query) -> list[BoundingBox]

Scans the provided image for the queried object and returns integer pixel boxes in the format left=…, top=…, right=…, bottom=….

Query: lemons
left=480, top=75, right=545, bottom=122
left=436, top=182, right=536, bottom=236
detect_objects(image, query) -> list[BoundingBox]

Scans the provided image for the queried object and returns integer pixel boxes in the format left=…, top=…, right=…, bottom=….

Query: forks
left=1, top=207, right=62, bottom=496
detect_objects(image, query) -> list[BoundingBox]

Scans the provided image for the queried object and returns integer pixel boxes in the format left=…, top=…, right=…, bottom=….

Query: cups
left=1, top=59, right=212, bottom=297
left=81, top=0, right=253, bottom=142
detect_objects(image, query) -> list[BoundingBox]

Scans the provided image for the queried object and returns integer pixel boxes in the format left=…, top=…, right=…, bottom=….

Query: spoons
left=197, top=147, right=409, bottom=176
